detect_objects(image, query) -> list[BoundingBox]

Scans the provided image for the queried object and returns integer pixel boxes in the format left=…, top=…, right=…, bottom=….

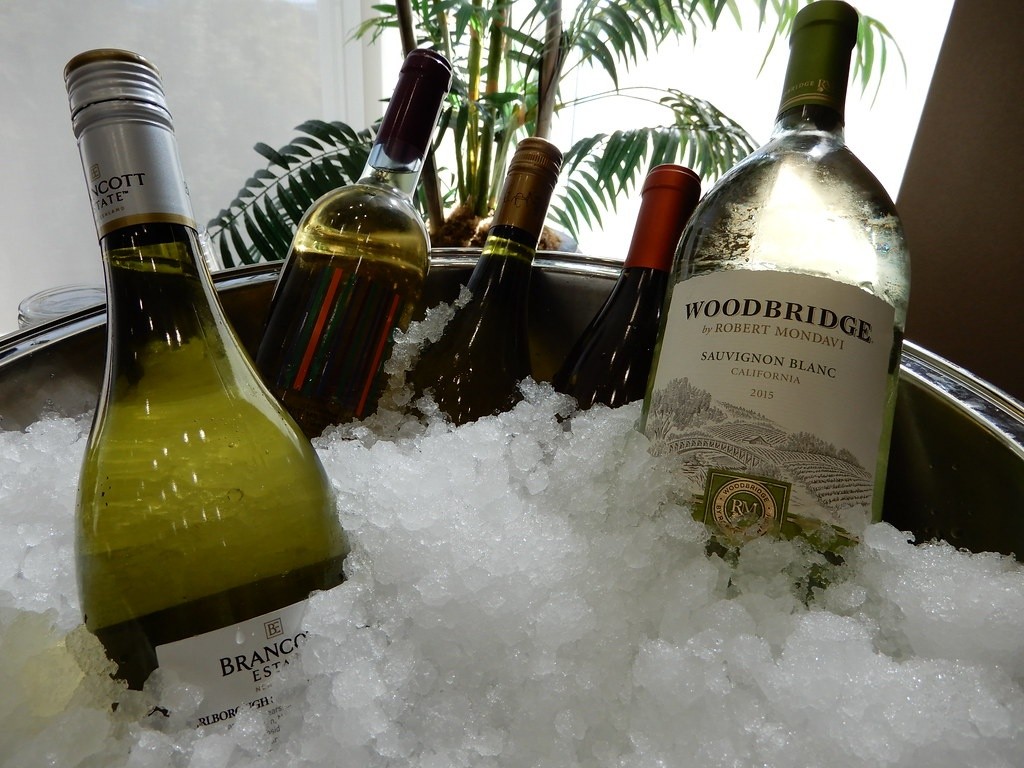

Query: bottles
left=543, top=158, right=698, bottom=433
left=58, top=46, right=352, bottom=768
left=634, top=0, right=910, bottom=630
left=373, top=126, right=562, bottom=438
left=238, top=48, right=464, bottom=450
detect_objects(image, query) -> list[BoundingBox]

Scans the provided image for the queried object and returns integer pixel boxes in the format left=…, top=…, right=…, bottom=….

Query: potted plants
left=203, top=0, right=907, bottom=272
